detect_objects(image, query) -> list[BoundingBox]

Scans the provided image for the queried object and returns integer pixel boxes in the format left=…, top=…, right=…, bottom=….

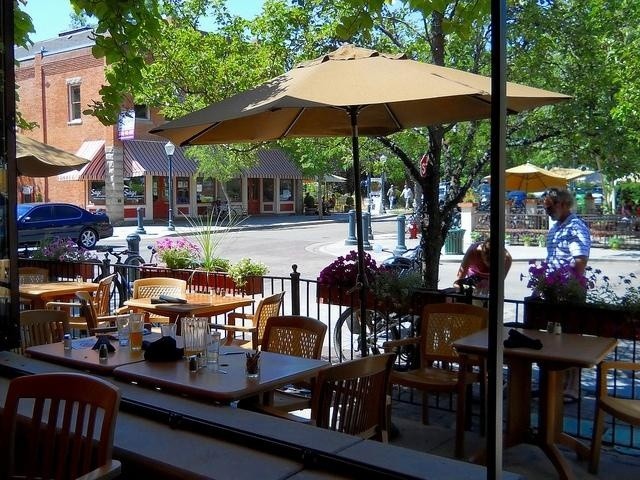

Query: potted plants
left=504, top=234, right=513, bottom=246
left=519, top=232, right=534, bottom=247
left=609, top=238, right=625, bottom=249
left=537, top=235, right=547, bottom=248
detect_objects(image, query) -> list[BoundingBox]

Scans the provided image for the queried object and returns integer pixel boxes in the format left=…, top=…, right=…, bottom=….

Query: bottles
left=64, top=334, right=73, bottom=351
left=99, top=344, right=109, bottom=360
left=546, top=322, right=562, bottom=335
left=188, top=352, right=206, bottom=373
left=210, top=287, right=225, bottom=297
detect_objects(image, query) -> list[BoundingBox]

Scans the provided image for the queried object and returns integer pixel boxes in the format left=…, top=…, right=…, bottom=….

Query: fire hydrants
left=409, top=219, right=418, bottom=239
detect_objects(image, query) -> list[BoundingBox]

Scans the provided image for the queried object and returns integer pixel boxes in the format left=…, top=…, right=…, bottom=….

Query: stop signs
left=419, top=152, right=428, bottom=177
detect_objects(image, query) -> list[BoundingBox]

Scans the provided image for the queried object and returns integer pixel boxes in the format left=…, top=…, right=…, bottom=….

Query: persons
left=387, top=184, right=397, bottom=210
left=592, top=188, right=605, bottom=211
left=326, top=194, right=336, bottom=210
left=400, top=185, right=413, bottom=209
left=453, top=237, right=511, bottom=308
left=635, top=199, right=640, bottom=232
left=624, top=200, right=634, bottom=234
left=526, top=186, right=590, bottom=405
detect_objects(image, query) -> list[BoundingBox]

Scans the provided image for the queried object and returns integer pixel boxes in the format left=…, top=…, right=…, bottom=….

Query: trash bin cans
left=445, top=229, right=466, bottom=255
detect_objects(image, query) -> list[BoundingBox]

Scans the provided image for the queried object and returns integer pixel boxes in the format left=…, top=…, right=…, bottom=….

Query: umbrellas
left=504, top=161, right=567, bottom=200
left=548, top=166, right=596, bottom=181
left=15, top=130, right=92, bottom=185
left=147, top=43, right=573, bottom=390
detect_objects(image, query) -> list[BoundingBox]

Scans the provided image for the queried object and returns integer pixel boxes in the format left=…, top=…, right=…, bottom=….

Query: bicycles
left=333, top=272, right=488, bottom=370
left=201, top=200, right=239, bottom=222
left=91, top=245, right=146, bottom=314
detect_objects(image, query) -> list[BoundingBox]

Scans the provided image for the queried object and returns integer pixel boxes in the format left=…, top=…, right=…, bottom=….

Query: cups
left=129, top=312, right=145, bottom=351
left=87, top=278, right=92, bottom=286
left=181, top=317, right=220, bottom=363
left=117, top=315, right=129, bottom=347
left=244, top=356, right=262, bottom=378
left=77, top=275, right=84, bottom=284
left=160, top=323, right=177, bottom=339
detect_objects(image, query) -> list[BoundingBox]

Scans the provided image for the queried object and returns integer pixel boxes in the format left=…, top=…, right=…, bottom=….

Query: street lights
left=379, top=155, right=387, bottom=215
left=165, top=141, right=178, bottom=230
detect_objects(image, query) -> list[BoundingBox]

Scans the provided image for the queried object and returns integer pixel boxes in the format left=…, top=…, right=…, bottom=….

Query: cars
left=474, top=183, right=606, bottom=214
left=411, top=183, right=448, bottom=210
left=15, top=202, right=114, bottom=250
left=280, top=189, right=291, bottom=200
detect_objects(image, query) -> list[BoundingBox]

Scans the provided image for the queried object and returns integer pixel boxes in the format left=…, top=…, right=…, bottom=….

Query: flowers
left=150, top=237, right=200, bottom=269
left=42, top=235, right=99, bottom=264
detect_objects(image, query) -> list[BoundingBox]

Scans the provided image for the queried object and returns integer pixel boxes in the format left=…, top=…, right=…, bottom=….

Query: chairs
left=384, top=304, right=489, bottom=458
left=1, top=259, right=285, bottom=376
left=2, top=372, right=122, bottom=480
left=261, top=315, right=327, bottom=412
left=271, top=353, right=398, bottom=444
left=588, top=359, right=639, bottom=478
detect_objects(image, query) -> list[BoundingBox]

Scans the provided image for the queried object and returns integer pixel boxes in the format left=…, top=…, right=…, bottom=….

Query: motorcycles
left=344, top=241, right=424, bottom=333
